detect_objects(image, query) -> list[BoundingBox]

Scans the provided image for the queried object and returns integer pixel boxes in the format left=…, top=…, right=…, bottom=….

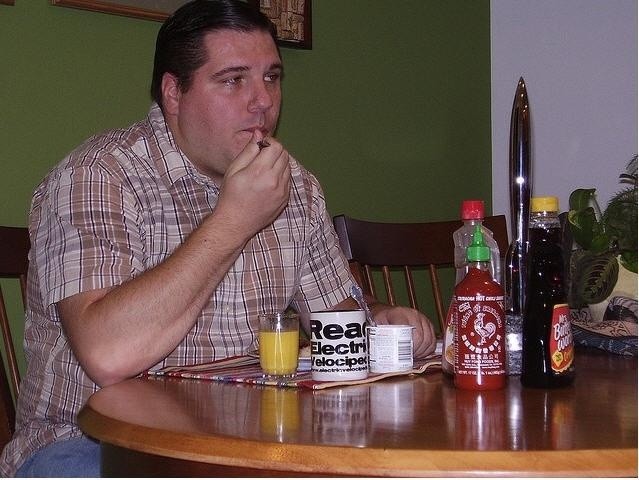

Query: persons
left=2, top=0, right=439, bottom=478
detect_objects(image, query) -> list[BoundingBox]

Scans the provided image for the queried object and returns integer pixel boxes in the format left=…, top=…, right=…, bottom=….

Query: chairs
left=0, top=226, right=32, bottom=458
left=333, top=215, right=510, bottom=338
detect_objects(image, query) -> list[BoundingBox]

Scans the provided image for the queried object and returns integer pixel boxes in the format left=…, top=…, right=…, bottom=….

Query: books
left=144, top=348, right=443, bottom=391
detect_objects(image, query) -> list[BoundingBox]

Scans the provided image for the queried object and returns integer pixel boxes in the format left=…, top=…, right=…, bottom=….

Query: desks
left=76, top=342, right=638, bottom=478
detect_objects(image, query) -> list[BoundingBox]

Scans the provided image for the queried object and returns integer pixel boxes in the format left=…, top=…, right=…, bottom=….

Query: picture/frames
left=52, top=0, right=312, bottom=50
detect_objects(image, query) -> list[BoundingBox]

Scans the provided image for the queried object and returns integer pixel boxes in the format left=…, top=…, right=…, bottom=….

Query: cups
left=258, top=314, right=300, bottom=379
left=310, top=309, right=416, bottom=383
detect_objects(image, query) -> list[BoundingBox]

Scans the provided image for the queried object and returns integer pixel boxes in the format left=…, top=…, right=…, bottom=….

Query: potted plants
left=558, top=153, right=638, bottom=346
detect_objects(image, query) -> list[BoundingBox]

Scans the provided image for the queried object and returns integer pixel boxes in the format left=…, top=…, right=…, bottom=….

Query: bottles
left=442, top=198, right=574, bottom=392
